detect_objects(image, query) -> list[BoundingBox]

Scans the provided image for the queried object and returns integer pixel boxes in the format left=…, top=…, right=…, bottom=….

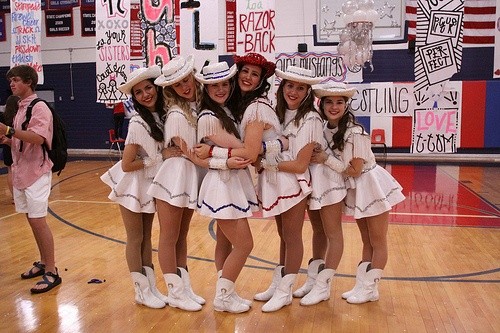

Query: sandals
left=20, top=261, right=46, bottom=279
left=30, top=267, right=62, bottom=293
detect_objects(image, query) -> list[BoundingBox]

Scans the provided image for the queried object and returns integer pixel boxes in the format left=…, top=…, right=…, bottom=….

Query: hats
left=195, top=61, right=236, bottom=84
left=274, top=65, right=320, bottom=86
left=235, top=53, right=275, bottom=78
left=153, top=54, right=194, bottom=86
left=313, top=81, right=357, bottom=99
left=118, top=65, right=160, bottom=95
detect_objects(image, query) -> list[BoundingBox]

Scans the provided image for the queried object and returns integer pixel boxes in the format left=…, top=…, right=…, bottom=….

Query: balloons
left=338, top=0, right=380, bottom=67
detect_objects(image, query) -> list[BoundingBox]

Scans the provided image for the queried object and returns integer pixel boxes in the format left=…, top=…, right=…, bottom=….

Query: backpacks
left=26, top=98, right=67, bottom=172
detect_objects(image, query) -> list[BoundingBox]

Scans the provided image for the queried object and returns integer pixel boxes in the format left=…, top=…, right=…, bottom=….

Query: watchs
left=5, top=126, right=15, bottom=139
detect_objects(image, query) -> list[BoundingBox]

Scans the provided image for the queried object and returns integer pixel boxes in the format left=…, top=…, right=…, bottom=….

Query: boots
left=254, top=264, right=286, bottom=302
left=341, top=261, right=371, bottom=299
left=213, top=276, right=250, bottom=313
left=300, top=264, right=336, bottom=305
left=218, top=270, right=253, bottom=306
left=293, top=258, right=324, bottom=297
left=347, top=263, right=385, bottom=305
left=130, top=266, right=166, bottom=309
left=163, top=268, right=202, bottom=311
left=143, top=263, right=168, bottom=303
left=261, top=268, right=298, bottom=313
left=178, top=265, right=206, bottom=305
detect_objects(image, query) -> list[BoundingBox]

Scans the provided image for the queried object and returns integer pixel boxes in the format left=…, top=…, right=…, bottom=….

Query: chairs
left=109, top=130, right=126, bottom=160
left=372, top=129, right=387, bottom=156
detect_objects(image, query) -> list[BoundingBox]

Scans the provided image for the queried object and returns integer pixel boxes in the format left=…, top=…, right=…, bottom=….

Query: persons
left=153, top=54, right=253, bottom=312
left=193, top=60, right=289, bottom=314
left=101, top=64, right=183, bottom=310
left=192, top=51, right=311, bottom=313
left=0, top=96, right=20, bottom=205
left=250, top=65, right=355, bottom=307
left=309, top=79, right=406, bottom=305
left=0, top=66, right=63, bottom=294
